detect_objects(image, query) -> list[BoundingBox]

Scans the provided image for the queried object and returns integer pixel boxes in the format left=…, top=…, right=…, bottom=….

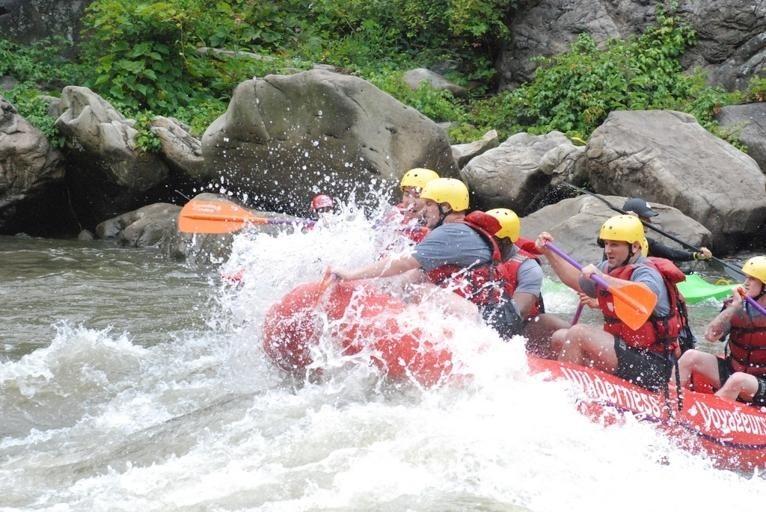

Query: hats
left=622, top=197, right=659, bottom=218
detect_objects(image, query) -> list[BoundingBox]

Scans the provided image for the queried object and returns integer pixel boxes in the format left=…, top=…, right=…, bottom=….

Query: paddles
left=543, top=241, right=657, bottom=331
left=177, top=198, right=429, bottom=234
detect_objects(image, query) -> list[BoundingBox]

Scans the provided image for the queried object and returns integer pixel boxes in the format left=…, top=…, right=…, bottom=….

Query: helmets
left=399, top=166, right=439, bottom=195
left=599, top=215, right=648, bottom=257
left=419, top=178, right=470, bottom=213
left=485, top=208, right=520, bottom=243
left=309, top=194, right=336, bottom=213
left=742, top=256, right=766, bottom=284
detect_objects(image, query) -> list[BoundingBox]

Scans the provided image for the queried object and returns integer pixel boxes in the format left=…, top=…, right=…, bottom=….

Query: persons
left=334, top=176, right=507, bottom=322
left=537, top=212, right=684, bottom=397
left=670, top=251, right=762, bottom=414
left=596, top=194, right=713, bottom=275
left=302, top=194, right=332, bottom=236
left=379, top=165, right=435, bottom=241
left=484, top=204, right=546, bottom=335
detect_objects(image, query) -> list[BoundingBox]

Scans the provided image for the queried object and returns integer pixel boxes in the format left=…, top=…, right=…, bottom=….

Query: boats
left=262, top=274, right=766, bottom=480
left=223, top=235, right=743, bottom=314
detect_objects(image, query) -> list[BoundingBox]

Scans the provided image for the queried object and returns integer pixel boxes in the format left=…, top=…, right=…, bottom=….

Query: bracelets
left=693, top=252, right=698, bottom=260
